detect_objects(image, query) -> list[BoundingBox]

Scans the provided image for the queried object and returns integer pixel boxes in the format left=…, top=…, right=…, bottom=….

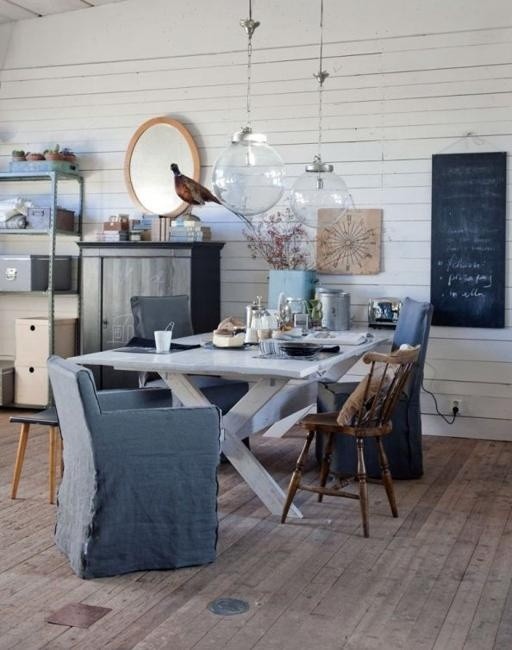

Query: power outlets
left=451, top=399, right=461, bottom=415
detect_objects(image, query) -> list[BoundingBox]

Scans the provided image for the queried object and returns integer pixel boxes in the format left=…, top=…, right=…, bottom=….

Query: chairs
left=45, top=354, right=221, bottom=580
left=129, top=294, right=251, bottom=452
left=280, top=344, right=422, bottom=538
left=317, top=297, right=434, bottom=479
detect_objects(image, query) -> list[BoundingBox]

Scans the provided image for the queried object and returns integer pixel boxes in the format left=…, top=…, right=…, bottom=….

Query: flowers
left=241, top=207, right=316, bottom=270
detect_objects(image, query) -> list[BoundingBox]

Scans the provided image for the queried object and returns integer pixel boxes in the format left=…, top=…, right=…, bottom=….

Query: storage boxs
left=0, top=361, right=14, bottom=407
left=14, top=317, right=79, bottom=410
left=26, top=207, right=76, bottom=230
left=0, top=254, right=72, bottom=292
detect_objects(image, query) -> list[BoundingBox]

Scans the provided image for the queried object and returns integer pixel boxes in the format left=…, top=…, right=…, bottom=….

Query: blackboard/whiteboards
left=431, top=152, right=506, bottom=328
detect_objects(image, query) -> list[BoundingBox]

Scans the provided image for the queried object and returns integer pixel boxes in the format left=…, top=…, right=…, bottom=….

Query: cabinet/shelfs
left=74, top=240, right=227, bottom=391
left=0, top=169, right=84, bottom=408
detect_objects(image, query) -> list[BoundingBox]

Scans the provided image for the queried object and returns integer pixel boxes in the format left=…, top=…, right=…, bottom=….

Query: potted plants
left=10, top=143, right=76, bottom=160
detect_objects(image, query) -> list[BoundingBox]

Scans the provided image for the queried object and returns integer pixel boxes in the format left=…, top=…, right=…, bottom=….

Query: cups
left=154, top=331, right=173, bottom=354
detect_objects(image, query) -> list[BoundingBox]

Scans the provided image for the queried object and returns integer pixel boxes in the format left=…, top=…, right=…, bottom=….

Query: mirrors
left=123, top=117, right=201, bottom=218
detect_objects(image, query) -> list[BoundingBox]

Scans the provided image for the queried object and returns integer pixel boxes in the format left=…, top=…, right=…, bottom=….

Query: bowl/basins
left=280, top=342, right=322, bottom=356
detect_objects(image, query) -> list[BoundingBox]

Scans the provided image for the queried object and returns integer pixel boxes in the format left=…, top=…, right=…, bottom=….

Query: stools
left=8, top=407, right=59, bottom=504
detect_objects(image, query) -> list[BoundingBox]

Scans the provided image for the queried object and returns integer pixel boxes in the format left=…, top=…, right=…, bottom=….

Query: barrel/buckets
left=320, top=292, right=350, bottom=331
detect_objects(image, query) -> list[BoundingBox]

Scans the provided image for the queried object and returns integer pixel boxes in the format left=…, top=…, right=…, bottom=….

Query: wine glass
left=274, top=304, right=289, bottom=332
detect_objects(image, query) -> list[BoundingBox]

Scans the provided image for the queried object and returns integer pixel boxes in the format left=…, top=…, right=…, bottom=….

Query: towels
left=0, top=197, right=34, bottom=229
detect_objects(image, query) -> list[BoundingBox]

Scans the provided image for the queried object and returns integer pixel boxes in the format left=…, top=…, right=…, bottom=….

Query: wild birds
left=170, top=163, right=251, bottom=223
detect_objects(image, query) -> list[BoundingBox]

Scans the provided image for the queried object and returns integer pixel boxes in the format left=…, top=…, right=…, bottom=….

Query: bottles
left=284, top=297, right=308, bottom=336
left=244, top=295, right=276, bottom=356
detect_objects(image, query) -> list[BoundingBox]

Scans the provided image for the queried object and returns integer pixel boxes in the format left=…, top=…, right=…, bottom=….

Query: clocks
left=315, top=207, right=383, bottom=277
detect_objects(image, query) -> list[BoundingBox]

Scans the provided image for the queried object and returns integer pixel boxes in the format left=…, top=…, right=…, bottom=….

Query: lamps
left=211, top=0, right=285, bottom=217
left=289, top=1, right=353, bottom=228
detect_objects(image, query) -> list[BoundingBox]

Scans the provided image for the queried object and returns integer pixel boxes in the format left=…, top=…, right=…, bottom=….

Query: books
left=301, top=332, right=375, bottom=346
left=95, top=230, right=128, bottom=242
left=151, top=218, right=211, bottom=243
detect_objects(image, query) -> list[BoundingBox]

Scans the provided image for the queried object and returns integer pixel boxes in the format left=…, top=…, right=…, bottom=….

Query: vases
left=267, top=270, right=317, bottom=313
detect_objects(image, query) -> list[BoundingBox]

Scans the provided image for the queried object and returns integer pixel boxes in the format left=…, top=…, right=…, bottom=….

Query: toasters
left=367, top=297, right=403, bottom=330
